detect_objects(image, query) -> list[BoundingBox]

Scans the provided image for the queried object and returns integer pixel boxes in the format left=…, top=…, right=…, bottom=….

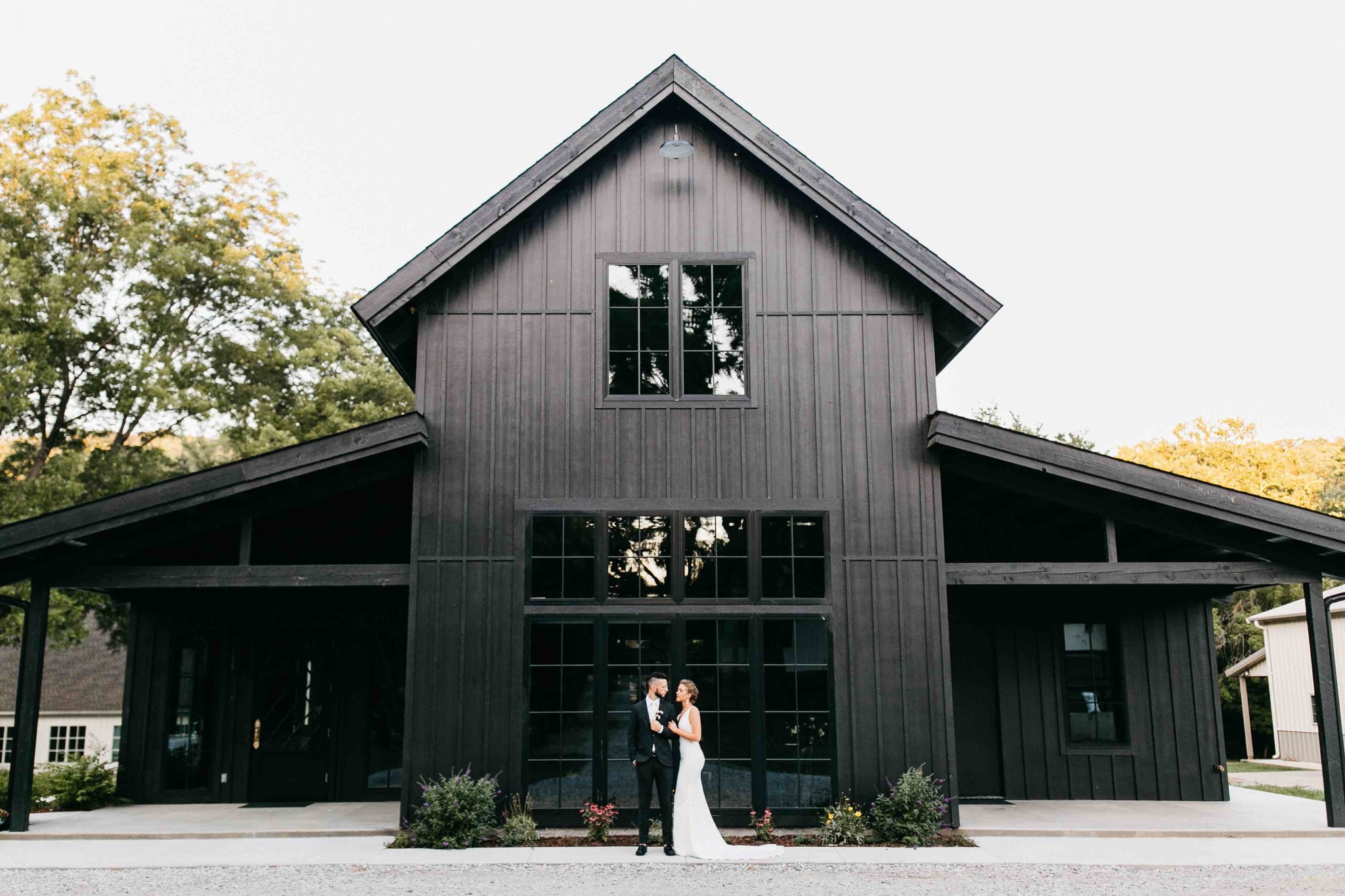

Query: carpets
left=238, top=802, right=315, bottom=808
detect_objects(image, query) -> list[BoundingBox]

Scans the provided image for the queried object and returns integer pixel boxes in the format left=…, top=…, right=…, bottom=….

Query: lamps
left=657, top=115, right=696, bottom=158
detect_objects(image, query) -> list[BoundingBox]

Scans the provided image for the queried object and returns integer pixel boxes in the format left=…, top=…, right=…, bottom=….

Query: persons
left=667, top=679, right=729, bottom=860
left=627, top=671, right=680, bottom=856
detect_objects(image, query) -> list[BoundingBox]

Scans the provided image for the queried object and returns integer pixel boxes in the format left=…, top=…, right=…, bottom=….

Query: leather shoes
left=664, top=844, right=675, bottom=856
left=636, top=845, right=648, bottom=856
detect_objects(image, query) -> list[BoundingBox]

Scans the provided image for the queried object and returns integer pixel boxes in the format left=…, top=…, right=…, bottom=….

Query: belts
left=650, top=752, right=658, bottom=758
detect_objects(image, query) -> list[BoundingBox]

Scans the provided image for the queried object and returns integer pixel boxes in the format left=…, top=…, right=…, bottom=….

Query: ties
left=651, top=701, right=656, bottom=722
